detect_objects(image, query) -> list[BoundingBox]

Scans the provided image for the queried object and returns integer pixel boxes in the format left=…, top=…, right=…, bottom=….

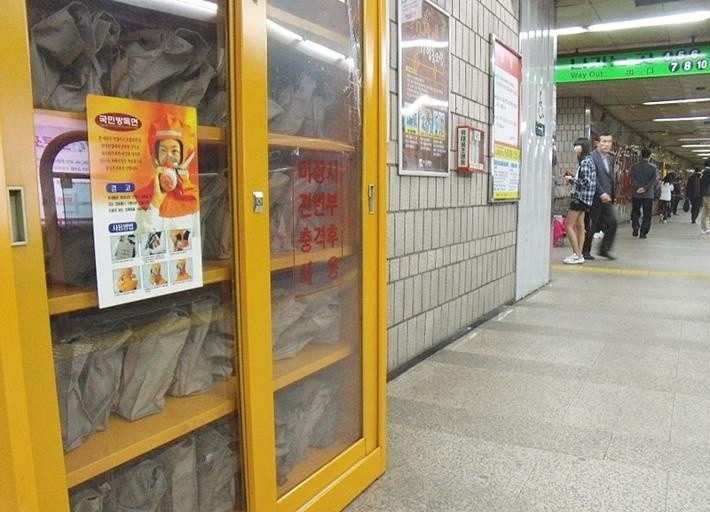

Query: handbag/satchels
left=198, top=161, right=233, bottom=261
left=267, top=148, right=345, bottom=256
left=274, top=369, right=344, bottom=488
left=28, top=3, right=229, bottom=126
left=275, top=265, right=345, bottom=359
left=681, top=201, right=690, bottom=211
left=266, top=56, right=342, bottom=140
left=55, top=227, right=93, bottom=283
left=52, top=288, right=240, bottom=509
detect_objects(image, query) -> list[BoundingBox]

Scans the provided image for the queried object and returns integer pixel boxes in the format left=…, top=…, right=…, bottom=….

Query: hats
left=147, top=114, right=194, bottom=169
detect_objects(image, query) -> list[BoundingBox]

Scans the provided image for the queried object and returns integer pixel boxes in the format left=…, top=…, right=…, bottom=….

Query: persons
left=701, top=159, right=710, bottom=234
left=563, top=137, right=597, bottom=264
left=583, top=131, right=617, bottom=259
left=630, top=149, right=658, bottom=238
left=653, top=166, right=704, bottom=223
left=133, top=111, right=197, bottom=231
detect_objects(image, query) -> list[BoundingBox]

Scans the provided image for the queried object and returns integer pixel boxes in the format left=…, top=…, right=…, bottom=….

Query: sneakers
left=632, top=213, right=709, bottom=239
left=562, top=250, right=618, bottom=266
